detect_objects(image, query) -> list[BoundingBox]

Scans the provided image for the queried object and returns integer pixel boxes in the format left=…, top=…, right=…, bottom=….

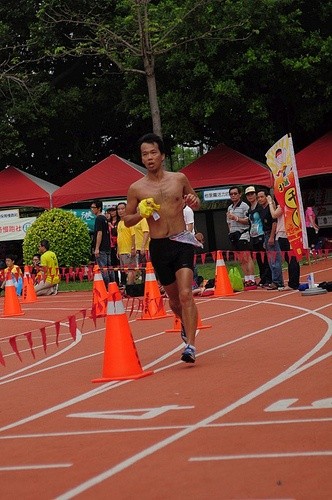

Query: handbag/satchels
left=228, top=231, right=241, bottom=243
left=228, top=267, right=244, bottom=292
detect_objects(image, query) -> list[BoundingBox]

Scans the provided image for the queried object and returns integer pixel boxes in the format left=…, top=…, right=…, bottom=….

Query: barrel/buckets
left=125, top=284, right=144, bottom=298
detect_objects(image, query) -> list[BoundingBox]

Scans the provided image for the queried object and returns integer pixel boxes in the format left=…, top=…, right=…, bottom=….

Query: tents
left=294, top=133, right=332, bottom=182
left=176, top=142, right=286, bottom=200
left=52, top=154, right=149, bottom=208
left=0, top=166, right=61, bottom=210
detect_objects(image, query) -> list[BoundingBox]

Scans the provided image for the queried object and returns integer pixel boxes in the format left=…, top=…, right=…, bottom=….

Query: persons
left=124, top=134, right=201, bottom=363
left=227, top=185, right=300, bottom=291
left=0, top=255, right=24, bottom=295
left=31, top=254, right=41, bottom=285
left=34, top=240, right=61, bottom=296
left=91, top=199, right=205, bottom=298
left=304, top=198, right=321, bottom=255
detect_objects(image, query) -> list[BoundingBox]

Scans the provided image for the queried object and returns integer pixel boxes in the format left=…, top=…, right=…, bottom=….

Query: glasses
left=90, top=206, right=96, bottom=208
left=229, top=192, right=239, bottom=195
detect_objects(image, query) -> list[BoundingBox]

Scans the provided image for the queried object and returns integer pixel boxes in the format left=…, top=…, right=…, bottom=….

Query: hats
left=244, top=186, right=256, bottom=194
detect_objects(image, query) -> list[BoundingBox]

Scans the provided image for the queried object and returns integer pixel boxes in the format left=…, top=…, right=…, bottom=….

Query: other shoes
left=278, top=286, right=293, bottom=291
left=181, top=322, right=187, bottom=344
left=243, top=280, right=257, bottom=287
left=265, top=282, right=278, bottom=290
left=180, top=347, right=196, bottom=364
left=52, top=283, right=59, bottom=295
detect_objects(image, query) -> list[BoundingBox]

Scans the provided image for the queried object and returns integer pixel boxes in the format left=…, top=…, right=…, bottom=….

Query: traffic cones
left=0, top=269, right=26, bottom=317
left=134, top=260, right=175, bottom=321
left=86, top=265, right=108, bottom=318
left=162, top=302, right=212, bottom=333
left=207, top=249, right=242, bottom=298
left=90, top=281, right=155, bottom=383
left=19, top=264, right=41, bottom=304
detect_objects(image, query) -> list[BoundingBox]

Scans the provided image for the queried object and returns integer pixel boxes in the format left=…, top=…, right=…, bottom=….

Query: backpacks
left=192, top=275, right=203, bottom=290
left=203, top=278, right=215, bottom=289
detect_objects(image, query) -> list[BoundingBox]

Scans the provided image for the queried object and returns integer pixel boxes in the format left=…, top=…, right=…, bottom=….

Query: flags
left=264, top=134, right=311, bottom=261
